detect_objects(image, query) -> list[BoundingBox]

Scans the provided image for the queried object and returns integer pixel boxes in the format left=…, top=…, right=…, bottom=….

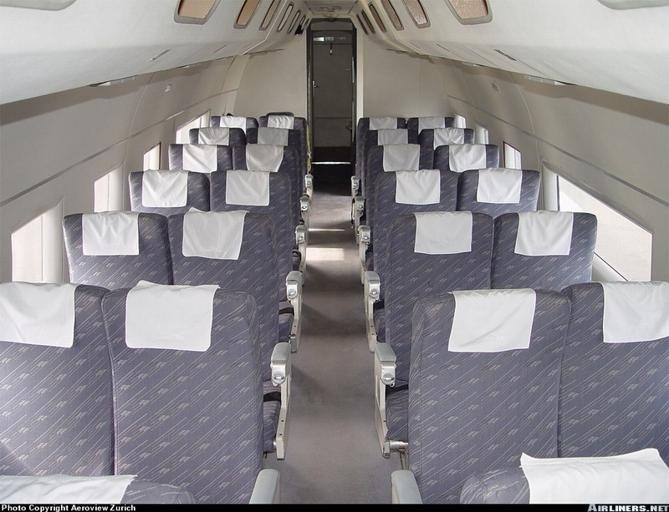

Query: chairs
left=352, top=115, right=669, bottom=506
left=1, top=110, right=310, bottom=503
